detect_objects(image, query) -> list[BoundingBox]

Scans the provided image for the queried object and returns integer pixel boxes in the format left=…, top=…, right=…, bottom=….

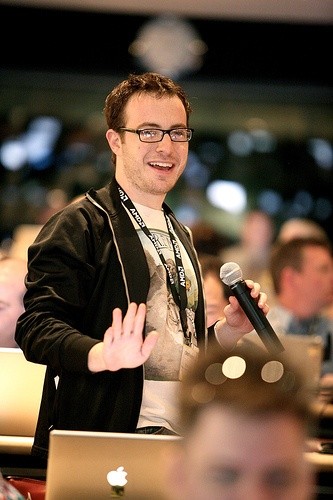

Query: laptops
left=46, top=430, right=189, bottom=500
left=235, top=331, right=322, bottom=398
left=0, top=348, right=47, bottom=436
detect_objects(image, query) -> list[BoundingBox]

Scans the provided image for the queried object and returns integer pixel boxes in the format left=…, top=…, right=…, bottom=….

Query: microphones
left=220, top=262, right=285, bottom=355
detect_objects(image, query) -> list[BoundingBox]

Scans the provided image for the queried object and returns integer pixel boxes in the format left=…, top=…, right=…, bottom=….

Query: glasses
left=119, top=127, right=195, bottom=143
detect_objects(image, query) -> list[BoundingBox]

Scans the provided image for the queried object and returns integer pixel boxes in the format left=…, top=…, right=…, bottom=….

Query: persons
left=174, top=341, right=311, bottom=500
left=0, top=189, right=333, bottom=376
left=14, top=72, right=269, bottom=458
